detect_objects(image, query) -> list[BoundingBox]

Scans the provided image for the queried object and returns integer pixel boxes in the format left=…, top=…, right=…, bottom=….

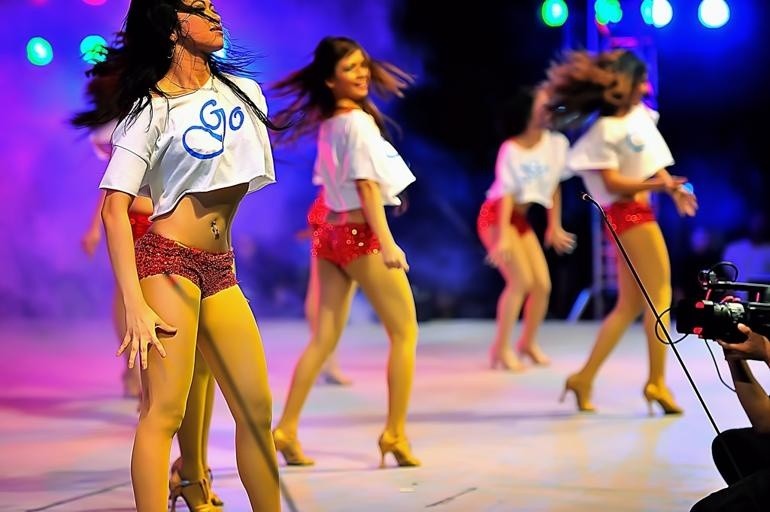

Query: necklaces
left=164, top=74, right=218, bottom=93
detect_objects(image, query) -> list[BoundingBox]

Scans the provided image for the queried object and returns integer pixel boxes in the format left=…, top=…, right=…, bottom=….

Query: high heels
left=169, top=456, right=224, bottom=512
left=491, top=342, right=551, bottom=372
left=316, top=362, right=352, bottom=385
left=560, top=374, right=592, bottom=411
left=644, top=384, right=682, bottom=415
left=273, top=428, right=314, bottom=465
left=379, top=432, right=419, bottom=467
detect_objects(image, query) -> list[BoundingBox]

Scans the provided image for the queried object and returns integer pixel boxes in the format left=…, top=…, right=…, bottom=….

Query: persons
left=540, top=47, right=699, bottom=418
left=303, top=188, right=351, bottom=384
left=689, top=295, right=770, bottom=512
left=264, top=36, right=421, bottom=469
left=98, top=0, right=282, bottom=512
left=169, top=347, right=223, bottom=512
left=475, top=86, right=576, bottom=374
left=68, top=71, right=154, bottom=397
